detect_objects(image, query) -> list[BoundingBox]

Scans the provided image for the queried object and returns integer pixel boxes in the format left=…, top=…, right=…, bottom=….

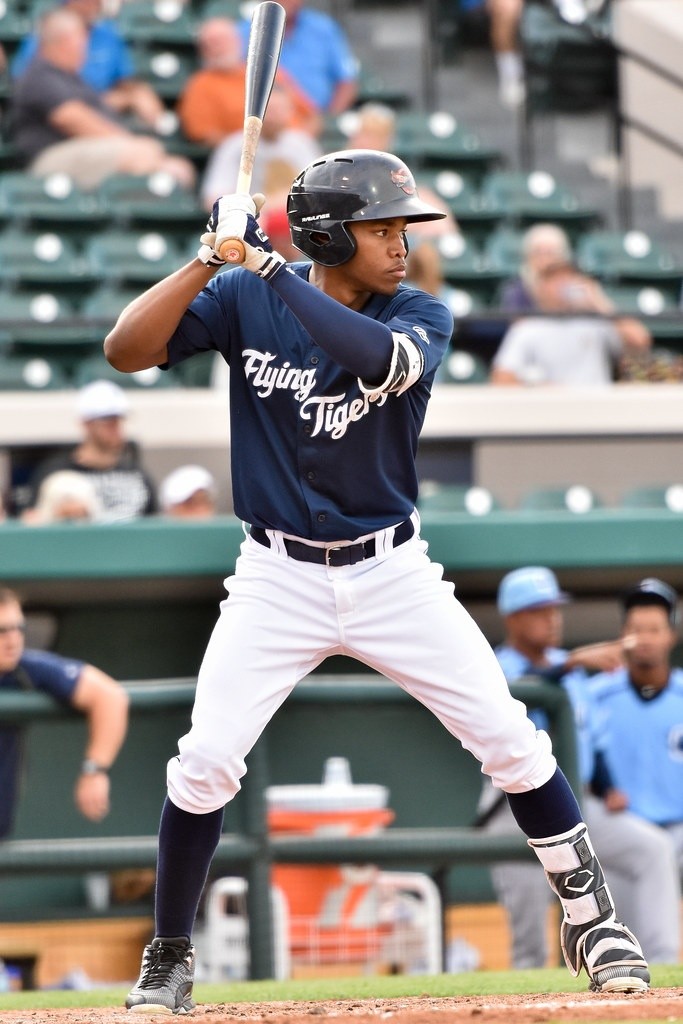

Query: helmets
left=622, top=578, right=677, bottom=628
left=284, top=148, right=447, bottom=266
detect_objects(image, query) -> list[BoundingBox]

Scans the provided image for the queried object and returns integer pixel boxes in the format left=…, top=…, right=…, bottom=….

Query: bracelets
left=81, top=762, right=109, bottom=776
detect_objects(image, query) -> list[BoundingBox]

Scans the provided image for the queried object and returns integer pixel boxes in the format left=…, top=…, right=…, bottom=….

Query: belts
left=249, top=517, right=414, bottom=570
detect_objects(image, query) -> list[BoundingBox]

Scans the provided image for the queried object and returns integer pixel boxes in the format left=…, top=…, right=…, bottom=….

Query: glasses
left=0, top=622, right=27, bottom=635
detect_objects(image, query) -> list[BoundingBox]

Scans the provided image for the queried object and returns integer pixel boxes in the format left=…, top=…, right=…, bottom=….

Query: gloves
left=201, top=210, right=286, bottom=282
left=198, top=189, right=266, bottom=268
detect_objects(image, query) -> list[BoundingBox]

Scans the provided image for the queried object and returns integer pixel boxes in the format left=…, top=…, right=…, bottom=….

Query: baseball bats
left=218, top=3, right=287, bottom=265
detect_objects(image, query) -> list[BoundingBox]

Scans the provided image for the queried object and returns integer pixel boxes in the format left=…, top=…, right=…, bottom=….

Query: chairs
left=1, top=1, right=683, bottom=394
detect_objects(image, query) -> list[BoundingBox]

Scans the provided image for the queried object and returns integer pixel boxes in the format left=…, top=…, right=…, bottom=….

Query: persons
left=0, top=0, right=683, bottom=382
left=102, top=150, right=649, bottom=1017
left=0, top=584, right=129, bottom=844
left=478, top=567, right=683, bottom=966
left=6, top=378, right=215, bottom=527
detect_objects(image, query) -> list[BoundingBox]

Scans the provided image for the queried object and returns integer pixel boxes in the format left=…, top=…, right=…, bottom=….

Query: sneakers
left=125, top=935, right=197, bottom=1015
left=583, top=945, right=650, bottom=994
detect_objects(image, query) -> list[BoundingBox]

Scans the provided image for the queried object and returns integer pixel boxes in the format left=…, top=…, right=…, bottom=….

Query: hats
left=497, top=563, right=568, bottom=621
left=74, top=379, right=129, bottom=424
left=156, top=464, right=217, bottom=507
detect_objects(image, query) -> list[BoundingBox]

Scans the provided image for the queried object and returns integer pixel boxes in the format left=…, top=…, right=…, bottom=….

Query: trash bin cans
left=260, top=782, right=397, bottom=982
